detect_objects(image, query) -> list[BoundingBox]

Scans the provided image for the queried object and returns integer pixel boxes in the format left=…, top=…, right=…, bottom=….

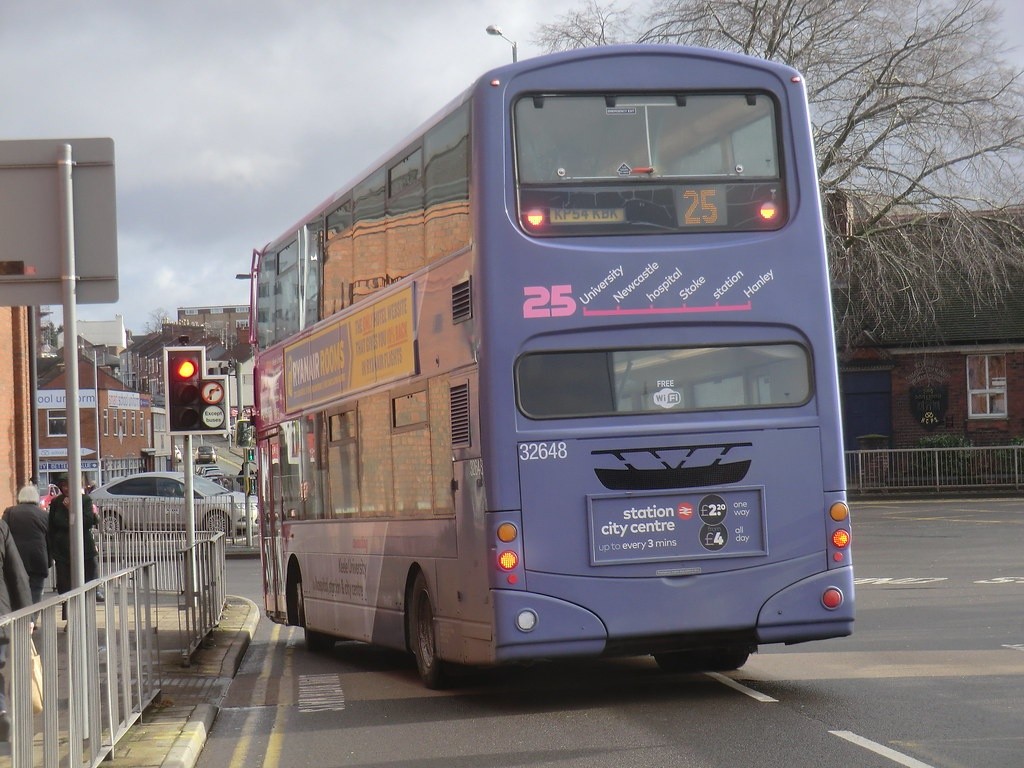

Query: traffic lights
left=246, top=447, right=255, bottom=463
left=163, top=346, right=206, bottom=436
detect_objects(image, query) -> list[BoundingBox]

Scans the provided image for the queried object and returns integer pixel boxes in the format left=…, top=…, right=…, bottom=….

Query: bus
left=229, top=44, right=858, bottom=693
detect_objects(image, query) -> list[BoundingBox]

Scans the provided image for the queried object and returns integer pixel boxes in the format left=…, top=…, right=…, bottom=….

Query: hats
left=58, top=473, right=68, bottom=482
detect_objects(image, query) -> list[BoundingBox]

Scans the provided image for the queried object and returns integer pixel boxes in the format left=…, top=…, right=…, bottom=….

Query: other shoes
left=34, top=626, right=38, bottom=631
left=64, top=624, right=67, bottom=632
left=96, top=590, right=105, bottom=602
left=62, top=612, right=66, bottom=620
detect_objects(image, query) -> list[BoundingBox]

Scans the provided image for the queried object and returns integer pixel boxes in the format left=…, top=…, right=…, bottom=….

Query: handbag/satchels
left=0, top=636, right=44, bottom=714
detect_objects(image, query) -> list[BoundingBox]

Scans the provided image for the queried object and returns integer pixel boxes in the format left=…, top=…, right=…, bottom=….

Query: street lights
left=484, top=23, right=518, bottom=63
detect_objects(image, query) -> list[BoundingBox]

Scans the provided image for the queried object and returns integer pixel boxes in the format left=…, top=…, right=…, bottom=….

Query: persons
left=165, top=484, right=179, bottom=497
left=213, top=462, right=258, bottom=494
left=39, top=472, right=105, bottom=635
left=2, top=484, right=52, bottom=639
left=0, top=516, right=36, bottom=743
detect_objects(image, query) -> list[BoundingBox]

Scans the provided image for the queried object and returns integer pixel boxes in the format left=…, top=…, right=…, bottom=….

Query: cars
left=174, top=444, right=183, bottom=463
left=196, top=464, right=259, bottom=496
left=37, top=483, right=63, bottom=512
left=87, top=471, right=259, bottom=540
left=195, top=445, right=218, bottom=464
left=223, top=425, right=236, bottom=441
